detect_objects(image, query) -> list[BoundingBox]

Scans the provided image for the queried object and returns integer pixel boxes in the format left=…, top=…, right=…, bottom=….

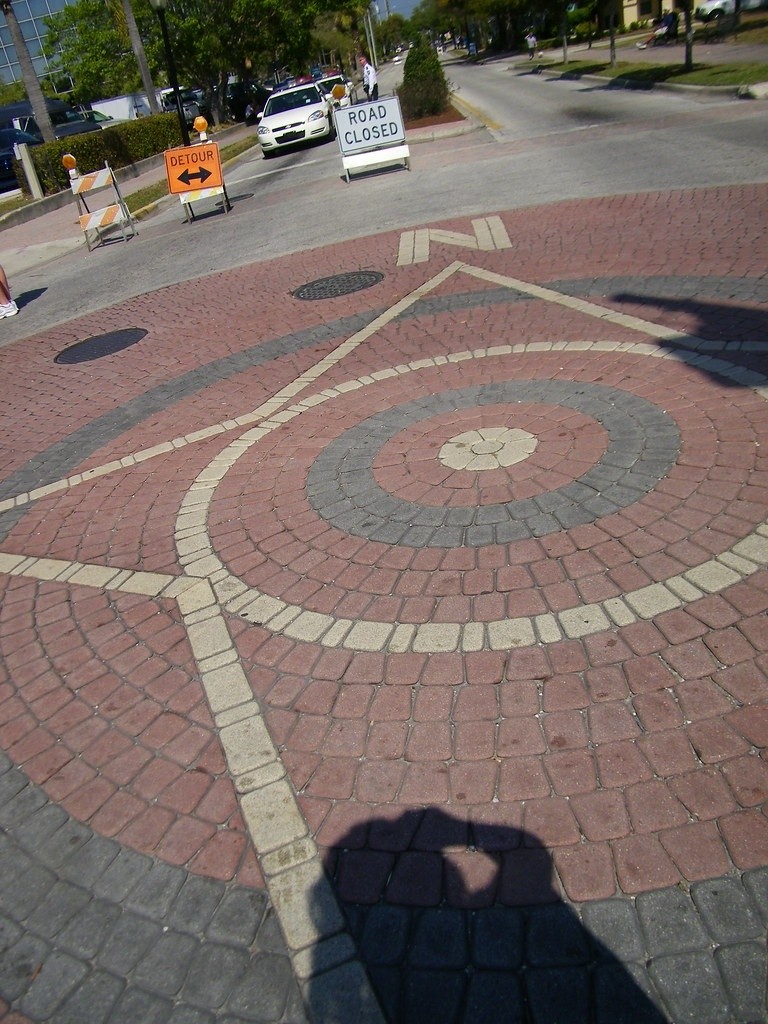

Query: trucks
left=161, top=86, right=202, bottom=127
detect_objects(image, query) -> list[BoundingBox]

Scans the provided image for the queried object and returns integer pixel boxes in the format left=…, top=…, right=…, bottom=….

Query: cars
left=310, top=67, right=358, bottom=112
left=694, top=1, right=768, bottom=25
left=257, top=74, right=341, bottom=159
left=0, top=128, right=44, bottom=180
left=67, top=109, right=131, bottom=129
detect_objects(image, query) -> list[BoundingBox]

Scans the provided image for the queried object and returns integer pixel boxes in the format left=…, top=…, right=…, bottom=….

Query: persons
left=456, top=36, right=464, bottom=49
left=525, top=31, right=537, bottom=59
left=359, top=56, right=378, bottom=101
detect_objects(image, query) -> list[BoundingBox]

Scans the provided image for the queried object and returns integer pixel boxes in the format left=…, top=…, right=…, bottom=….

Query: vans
left=0, top=98, right=102, bottom=142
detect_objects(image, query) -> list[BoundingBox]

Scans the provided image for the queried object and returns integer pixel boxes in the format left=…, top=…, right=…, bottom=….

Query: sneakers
left=0, top=301, right=19, bottom=320
